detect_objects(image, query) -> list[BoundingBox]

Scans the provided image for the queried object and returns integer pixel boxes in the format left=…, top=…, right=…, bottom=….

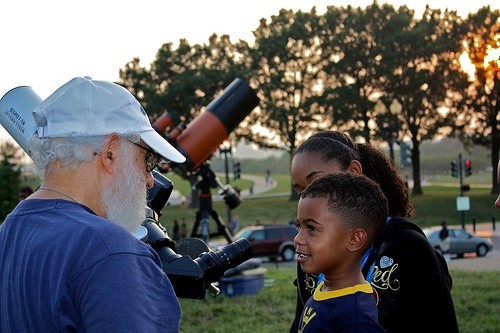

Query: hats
left=30, top=75, right=187, bottom=164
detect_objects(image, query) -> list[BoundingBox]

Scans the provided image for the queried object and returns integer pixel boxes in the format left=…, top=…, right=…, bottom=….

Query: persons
left=265, top=169, right=274, bottom=188
left=232, top=215, right=240, bottom=235
left=180, top=218, right=186, bottom=239
left=248, top=182, right=254, bottom=195
left=200, top=214, right=210, bottom=246
left=294, top=173, right=389, bottom=333
left=437, top=219, right=451, bottom=266
left=172, top=219, right=180, bottom=242
left=0, top=77, right=186, bottom=333
left=290, top=131, right=461, bottom=333
left=247, top=217, right=279, bottom=226
left=493, top=160, right=500, bottom=210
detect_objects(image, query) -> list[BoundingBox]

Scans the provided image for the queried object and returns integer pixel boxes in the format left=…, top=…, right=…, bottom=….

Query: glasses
left=122, top=136, right=161, bottom=172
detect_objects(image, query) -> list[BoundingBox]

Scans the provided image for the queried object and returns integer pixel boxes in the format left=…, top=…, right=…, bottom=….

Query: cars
left=419, top=225, right=494, bottom=257
left=164, top=189, right=186, bottom=207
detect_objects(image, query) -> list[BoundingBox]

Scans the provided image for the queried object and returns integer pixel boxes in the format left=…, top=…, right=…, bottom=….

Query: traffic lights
left=463, top=158, right=472, bottom=177
left=400, top=142, right=413, bottom=169
left=450, top=159, right=458, bottom=178
left=234, top=162, right=241, bottom=180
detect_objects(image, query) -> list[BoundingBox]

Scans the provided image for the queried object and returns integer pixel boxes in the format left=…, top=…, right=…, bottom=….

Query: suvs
left=210, top=224, right=299, bottom=261
